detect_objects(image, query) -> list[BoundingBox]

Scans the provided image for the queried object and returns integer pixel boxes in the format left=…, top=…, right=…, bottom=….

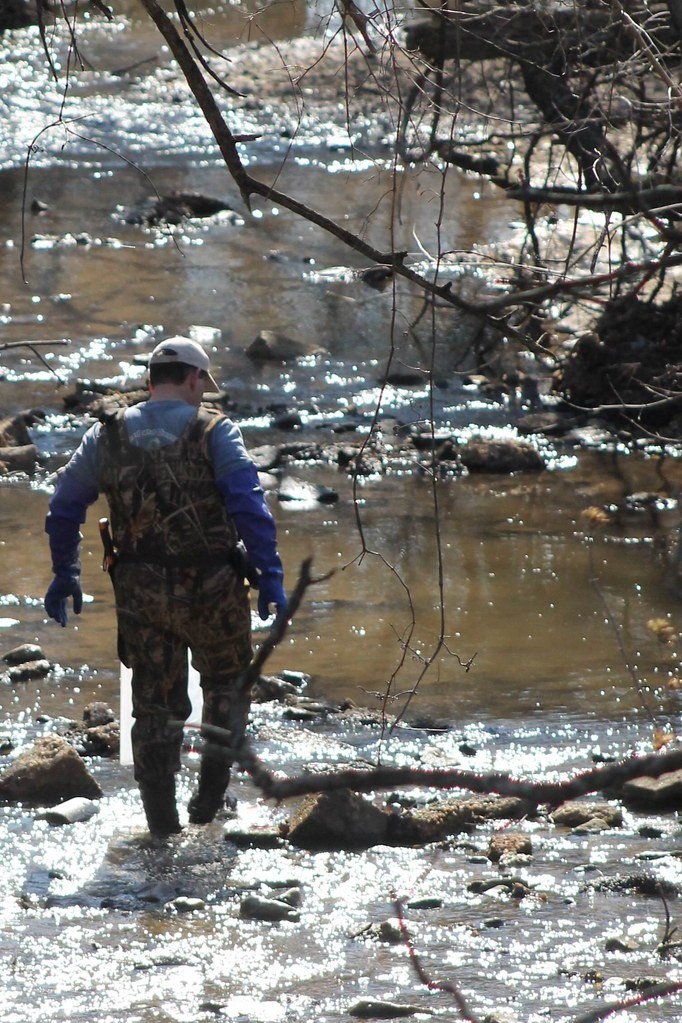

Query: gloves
left=44, top=471, right=98, bottom=629
left=215, top=464, right=289, bottom=621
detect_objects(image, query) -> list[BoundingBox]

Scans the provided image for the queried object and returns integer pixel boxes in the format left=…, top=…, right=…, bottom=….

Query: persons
left=44, top=336, right=289, bottom=838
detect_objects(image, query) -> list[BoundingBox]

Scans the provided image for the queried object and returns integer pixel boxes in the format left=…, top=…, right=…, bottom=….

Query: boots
left=188, top=761, right=237, bottom=826
left=140, top=791, right=176, bottom=838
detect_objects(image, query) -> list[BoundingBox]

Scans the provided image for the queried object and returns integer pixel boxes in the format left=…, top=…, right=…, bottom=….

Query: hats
left=149, top=333, right=219, bottom=394
